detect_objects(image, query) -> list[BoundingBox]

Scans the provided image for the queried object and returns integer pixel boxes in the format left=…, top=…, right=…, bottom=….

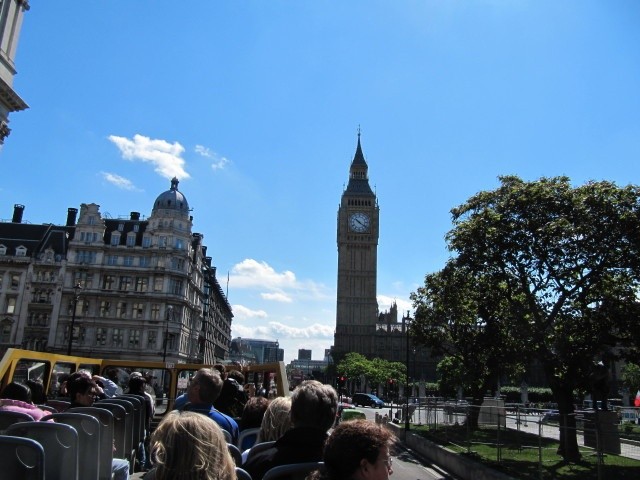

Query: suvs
left=352, top=393, right=384, bottom=409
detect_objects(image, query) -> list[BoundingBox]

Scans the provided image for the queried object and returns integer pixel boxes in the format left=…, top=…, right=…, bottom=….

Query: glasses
left=375, top=459, right=393, bottom=466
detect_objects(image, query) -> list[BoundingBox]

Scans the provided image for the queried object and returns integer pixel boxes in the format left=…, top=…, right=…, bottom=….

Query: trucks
left=583, top=397, right=637, bottom=425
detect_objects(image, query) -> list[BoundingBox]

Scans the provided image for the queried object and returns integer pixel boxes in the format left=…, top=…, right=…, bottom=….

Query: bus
left=287, top=368, right=304, bottom=391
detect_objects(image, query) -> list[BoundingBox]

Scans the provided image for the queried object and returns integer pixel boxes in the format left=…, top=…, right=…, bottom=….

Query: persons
left=150, top=375, right=163, bottom=407
left=256, top=380, right=267, bottom=396
left=64, top=376, right=130, bottom=480
left=57, top=371, right=106, bottom=400
left=125, top=376, right=151, bottom=471
left=106, top=368, right=120, bottom=386
left=244, top=378, right=338, bottom=480
left=442, top=400, right=454, bottom=424
left=245, top=382, right=256, bottom=395
left=176, top=367, right=239, bottom=449
left=144, top=409, right=239, bottom=480
left=306, top=418, right=393, bottom=479
left=241, top=395, right=269, bottom=429
left=23, top=379, right=50, bottom=406
left=92, top=374, right=118, bottom=397
left=241, top=396, right=292, bottom=463
left=267, top=374, right=275, bottom=399
left=125, top=371, right=156, bottom=419
left=212, top=364, right=239, bottom=419
left=0, top=382, right=55, bottom=423
left=227, top=369, right=244, bottom=398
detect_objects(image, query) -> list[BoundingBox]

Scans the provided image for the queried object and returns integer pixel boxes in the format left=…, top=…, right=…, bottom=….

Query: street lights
left=164, top=306, right=172, bottom=361
left=67, top=282, right=83, bottom=356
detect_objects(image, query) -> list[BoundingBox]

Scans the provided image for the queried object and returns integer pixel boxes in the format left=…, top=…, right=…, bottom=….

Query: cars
left=457, top=399, right=471, bottom=406
left=542, top=410, right=564, bottom=422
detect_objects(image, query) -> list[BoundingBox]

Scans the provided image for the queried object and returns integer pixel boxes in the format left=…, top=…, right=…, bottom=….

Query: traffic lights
left=388, top=379, right=392, bottom=384
left=340, top=376, right=345, bottom=387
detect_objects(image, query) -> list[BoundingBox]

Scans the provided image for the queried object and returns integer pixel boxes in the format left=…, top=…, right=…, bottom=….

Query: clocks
left=349, top=211, right=370, bottom=234
